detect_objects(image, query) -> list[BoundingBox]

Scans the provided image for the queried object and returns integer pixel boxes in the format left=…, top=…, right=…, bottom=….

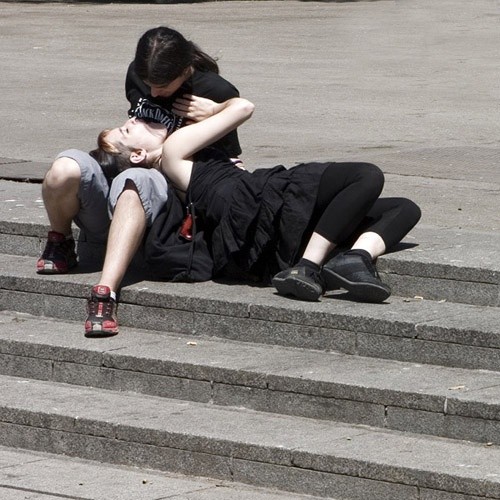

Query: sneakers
left=85, top=285, right=119, bottom=335
left=36, top=231, right=77, bottom=273
left=320, top=252, right=391, bottom=302
left=273, top=265, right=322, bottom=300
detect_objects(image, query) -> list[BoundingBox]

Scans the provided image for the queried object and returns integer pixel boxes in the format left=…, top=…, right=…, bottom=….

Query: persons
left=89, top=93, right=421, bottom=301
left=36, top=27, right=241, bottom=335
